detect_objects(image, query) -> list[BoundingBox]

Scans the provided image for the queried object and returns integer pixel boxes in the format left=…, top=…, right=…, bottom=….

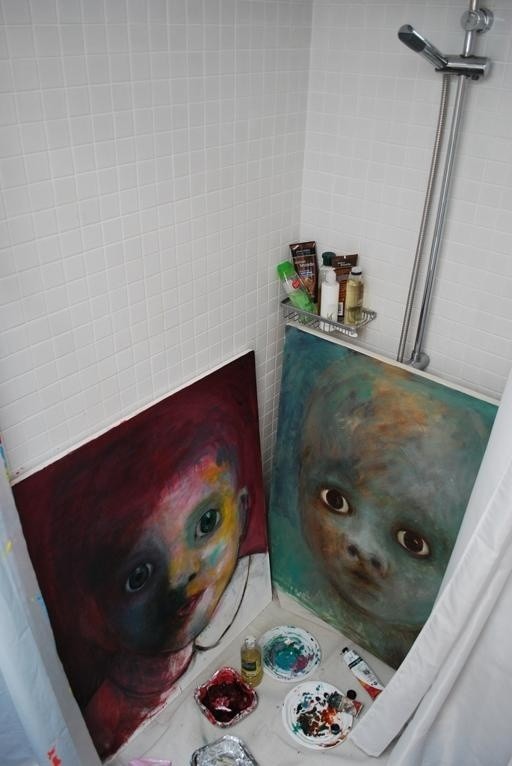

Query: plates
left=257, top=624, right=322, bottom=684
left=282, top=681, right=354, bottom=750
